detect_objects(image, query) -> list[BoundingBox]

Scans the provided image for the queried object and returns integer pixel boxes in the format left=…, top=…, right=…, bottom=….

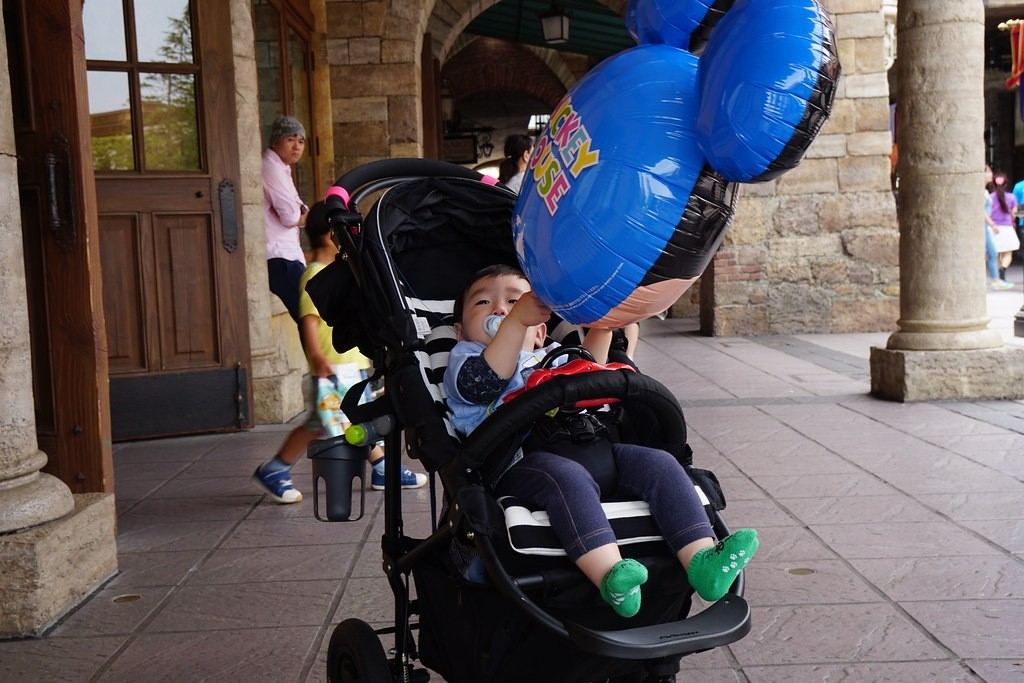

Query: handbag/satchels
left=318, top=377, right=366, bottom=437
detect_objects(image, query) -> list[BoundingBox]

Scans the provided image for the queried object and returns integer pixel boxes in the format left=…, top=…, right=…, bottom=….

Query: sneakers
left=371, top=467, right=428, bottom=490
left=250, top=463, right=302, bottom=503
left=993, top=280, right=1014, bottom=290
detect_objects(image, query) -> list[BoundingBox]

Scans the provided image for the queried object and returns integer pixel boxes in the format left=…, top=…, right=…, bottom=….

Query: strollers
left=305, top=154, right=757, bottom=683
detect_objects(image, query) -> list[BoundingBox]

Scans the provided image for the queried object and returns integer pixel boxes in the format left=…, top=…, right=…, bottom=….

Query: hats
left=269, top=116, right=306, bottom=146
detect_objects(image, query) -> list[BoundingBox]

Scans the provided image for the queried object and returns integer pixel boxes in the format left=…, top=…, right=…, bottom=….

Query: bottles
left=345, top=423, right=385, bottom=447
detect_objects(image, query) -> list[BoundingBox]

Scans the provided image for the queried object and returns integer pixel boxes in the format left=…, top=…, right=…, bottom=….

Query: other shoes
left=1000, top=267, right=1006, bottom=280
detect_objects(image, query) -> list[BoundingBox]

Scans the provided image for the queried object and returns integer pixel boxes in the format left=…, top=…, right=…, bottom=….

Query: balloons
left=697, top=0, right=841, bottom=185
left=625, top=0, right=735, bottom=53
left=513, top=41, right=739, bottom=329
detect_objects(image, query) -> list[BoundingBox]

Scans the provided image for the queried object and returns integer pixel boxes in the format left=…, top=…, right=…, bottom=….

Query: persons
left=261, top=115, right=309, bottom=326
left=247, top=196, right=427, bottom=501
left=442, top=263, right=759, bottom=619
left=499, top=134, right=534, bottom=195
left=989, top=172, right=1018, bottom=280
left=1011, top=180, right=1024, bottom=248
left=983, top=167, right=1013, bottom=289
